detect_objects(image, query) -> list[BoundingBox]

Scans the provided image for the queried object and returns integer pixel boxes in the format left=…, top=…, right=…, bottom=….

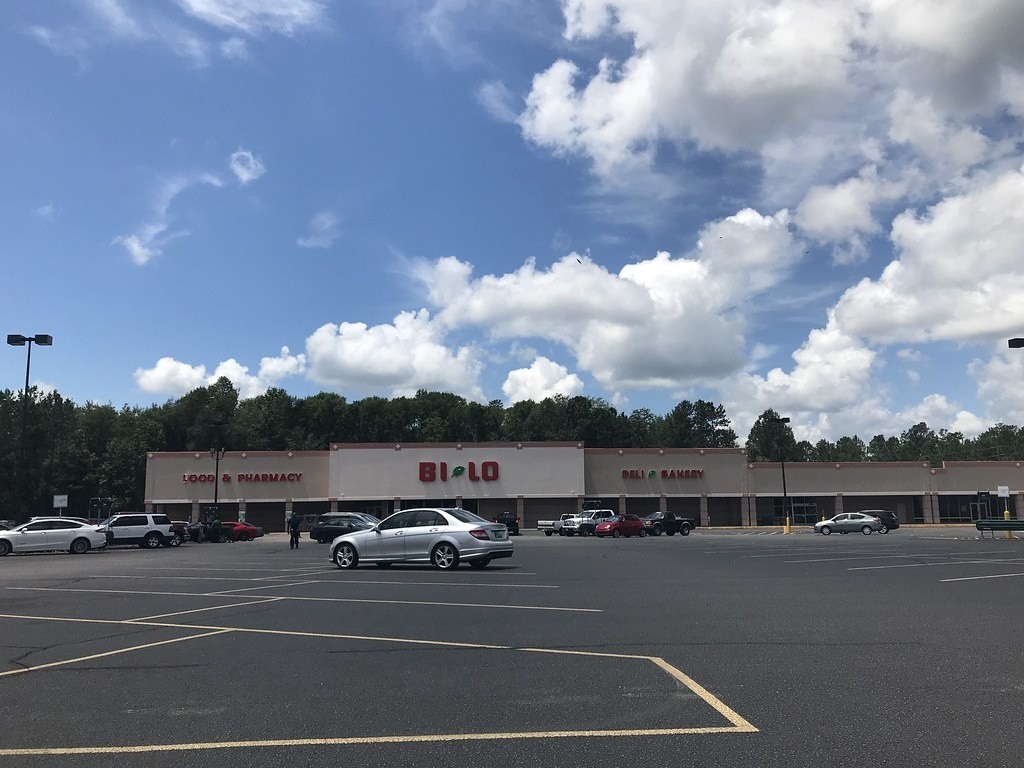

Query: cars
left=328, top=507, right=514, bottom=570
left=814, top=512, right=884, bottom=535
left=593, top=514, right=647, bottom=538
left=0, top=521, right=264, bottom=544
left=309, top=512, right=383, bottom=544
left=0, top=518, right=107, bottom=557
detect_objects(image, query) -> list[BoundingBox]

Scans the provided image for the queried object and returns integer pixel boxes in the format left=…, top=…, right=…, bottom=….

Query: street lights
left=210, top=447, right=225, bottom=518
left=768, top=417, right=792, bottom=535
left=6, top=333, right=54, bottom=530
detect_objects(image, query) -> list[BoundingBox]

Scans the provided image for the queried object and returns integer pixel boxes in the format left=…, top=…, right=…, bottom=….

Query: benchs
left=976, top=520, right=1024, bottom=539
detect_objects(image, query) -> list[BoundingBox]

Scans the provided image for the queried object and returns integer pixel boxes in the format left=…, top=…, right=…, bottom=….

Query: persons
left=287, top=512, right=301, bottom=550
left=212, top=517, right=221, bottom=542
left=197, top=519, right=204, bottom=543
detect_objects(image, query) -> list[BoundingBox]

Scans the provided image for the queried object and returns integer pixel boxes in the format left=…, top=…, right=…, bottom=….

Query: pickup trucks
left=537, top=513, right=585, bottom=536
left=643, top=510, right=696, bottom=536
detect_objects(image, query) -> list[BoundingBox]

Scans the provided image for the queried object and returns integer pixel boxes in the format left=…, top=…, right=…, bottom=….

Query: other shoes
left=299, top=537, right=301, bottom=538
left=290, top=547, right=293, bottom=549
left=296, top=544, right=298, bottom=548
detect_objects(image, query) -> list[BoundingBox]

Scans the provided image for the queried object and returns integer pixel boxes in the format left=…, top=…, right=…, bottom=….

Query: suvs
left=847, top=509, right=899, bottom=534
left=562, top=509, right=616, bottom=537
left=96, top=511, right=175, bottom=549
left=493, top=511, right=520, bottom=535
